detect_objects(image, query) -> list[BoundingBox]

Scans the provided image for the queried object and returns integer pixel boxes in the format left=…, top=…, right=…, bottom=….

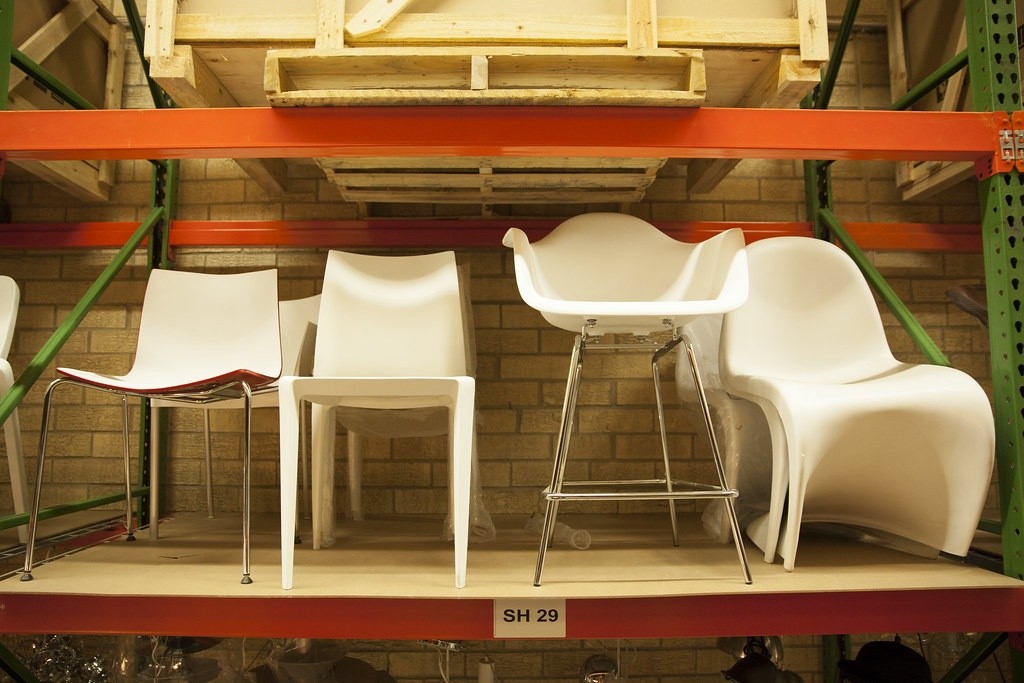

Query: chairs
left=713, top=235, right=996, bottom=571
left=21, top=269, right=282, bottom=585
left=278, top=250, right=474, bottom=591
left=149, top=295, right=322, bottom=543
left=0, top=274, right=29, bottom=543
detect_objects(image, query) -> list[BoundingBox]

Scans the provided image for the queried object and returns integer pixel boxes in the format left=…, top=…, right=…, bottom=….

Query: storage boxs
left=883, top=0, right=1024, bottom=203
left=5, top=3, right=127, bottom=205
left=143, top=0, right=830, bottom=195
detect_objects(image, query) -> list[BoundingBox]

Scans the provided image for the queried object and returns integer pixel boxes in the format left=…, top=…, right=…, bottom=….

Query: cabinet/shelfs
left=0, top=0, right=1024, bottom=641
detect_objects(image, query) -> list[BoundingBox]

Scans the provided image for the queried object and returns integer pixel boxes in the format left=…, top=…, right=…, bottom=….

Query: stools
left=502, top=211, right=753, bottom=588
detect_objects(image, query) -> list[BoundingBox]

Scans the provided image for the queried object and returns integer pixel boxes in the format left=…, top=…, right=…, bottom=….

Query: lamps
left=0, top=631, right=934, bottom=683
